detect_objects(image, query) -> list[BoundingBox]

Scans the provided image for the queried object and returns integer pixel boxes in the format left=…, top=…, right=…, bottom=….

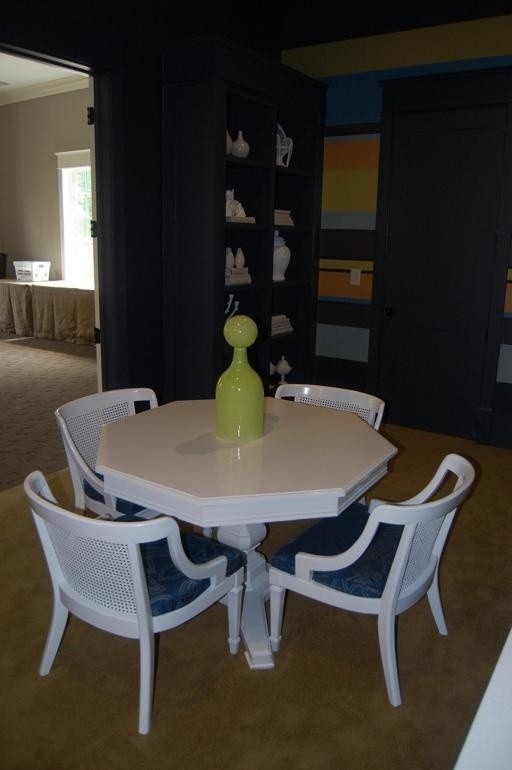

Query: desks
left=96, top=397, right=399, bottom=671
left=0, top=278, right=96, bottom=347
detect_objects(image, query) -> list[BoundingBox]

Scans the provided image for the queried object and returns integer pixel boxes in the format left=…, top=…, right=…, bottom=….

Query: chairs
left=276, top=383, right=386, bottom=506
left=54, top=390, right=213, bottom=540
left=270, top=454, right=477, bottom=706
left=25, top=469, right=246, bottom=732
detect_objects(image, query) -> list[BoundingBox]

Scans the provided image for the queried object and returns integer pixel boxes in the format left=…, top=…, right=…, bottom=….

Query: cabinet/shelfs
left=157, top=30, right=332, bottom=408
